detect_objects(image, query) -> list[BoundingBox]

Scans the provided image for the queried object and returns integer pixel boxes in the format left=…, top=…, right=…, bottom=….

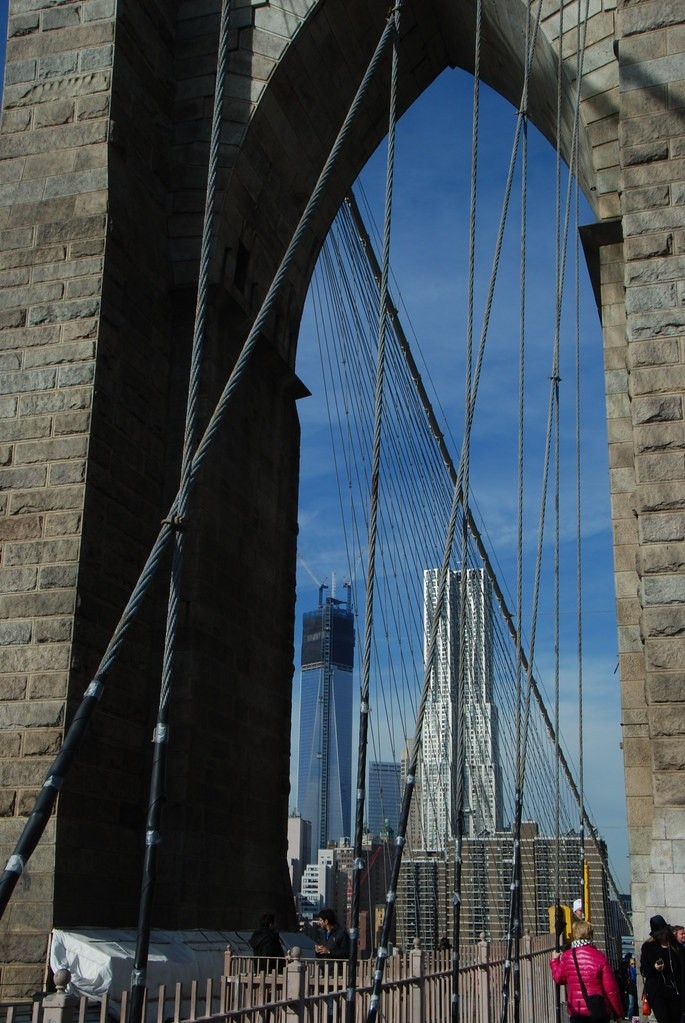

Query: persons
left=622, top=952, right=636, bottom=1019
left=432, top=936, right=453, bottom=994
left=314, top=907, right=351, bottom=1023
left=550, top=920, right=624, bottom=1023
left=639, top=915, right=685, bottom=1023
left=250, top=914, right=287, bottom=1023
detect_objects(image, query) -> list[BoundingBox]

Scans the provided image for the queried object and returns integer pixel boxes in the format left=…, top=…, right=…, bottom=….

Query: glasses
left=630, top=962, right=636, bottom=966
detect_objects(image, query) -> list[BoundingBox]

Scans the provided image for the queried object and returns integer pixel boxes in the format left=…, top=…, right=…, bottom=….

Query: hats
left=573, top=899, right=582, bottom=914
left=649, top=915, right=670, bottom=936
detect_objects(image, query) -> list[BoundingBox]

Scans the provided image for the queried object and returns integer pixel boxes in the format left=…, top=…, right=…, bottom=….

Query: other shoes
left=625, top=1017, right=631, bottom=1021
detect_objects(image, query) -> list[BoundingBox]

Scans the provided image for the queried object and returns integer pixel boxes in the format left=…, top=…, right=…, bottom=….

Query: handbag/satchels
left=641, top=994, right=651, bottom=1015
left=587, top=994, right=610, bottom=1023
